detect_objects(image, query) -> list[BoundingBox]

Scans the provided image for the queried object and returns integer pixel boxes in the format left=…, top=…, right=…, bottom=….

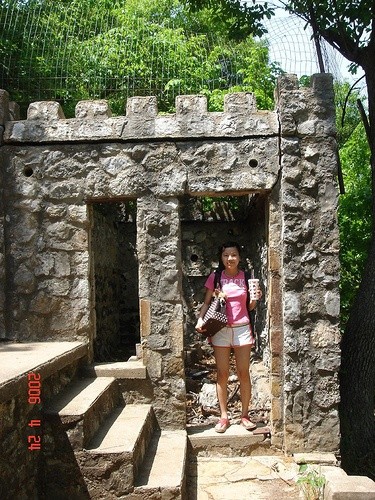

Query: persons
left=195, top=242, right=263, bottom=432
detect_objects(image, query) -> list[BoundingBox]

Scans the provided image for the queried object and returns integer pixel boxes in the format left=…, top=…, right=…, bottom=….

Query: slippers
left=215, top=418, right=230, bottom=433
left=240, top=417, right=256, bottom=430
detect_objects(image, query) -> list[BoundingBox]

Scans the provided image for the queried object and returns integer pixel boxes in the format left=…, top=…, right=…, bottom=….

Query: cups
left=248, top=278, right=260, bottom=301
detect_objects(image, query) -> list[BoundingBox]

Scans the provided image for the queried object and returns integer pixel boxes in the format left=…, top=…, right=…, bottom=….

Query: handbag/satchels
left=195, top=272, right=228, bottom=337
